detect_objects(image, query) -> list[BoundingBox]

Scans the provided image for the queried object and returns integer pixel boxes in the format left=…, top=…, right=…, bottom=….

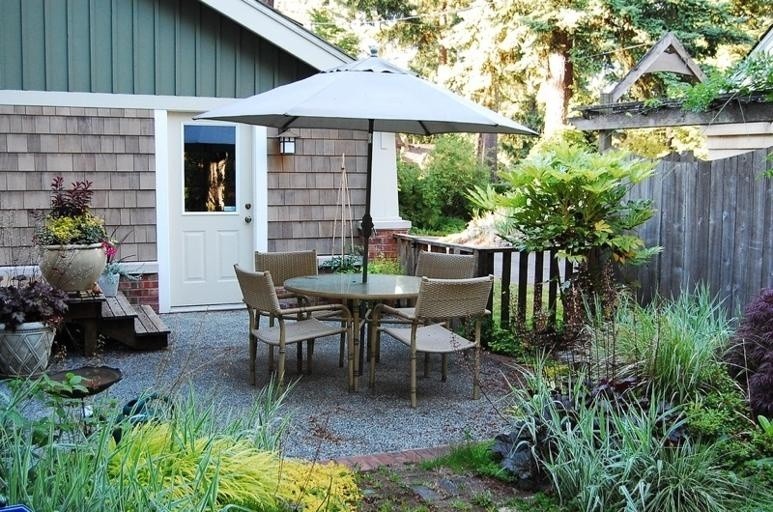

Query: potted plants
left=0, top=271, right=71, bottom=379
left=95, top=222, right=143, bottom=298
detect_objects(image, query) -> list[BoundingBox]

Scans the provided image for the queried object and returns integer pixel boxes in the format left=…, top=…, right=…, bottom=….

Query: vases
left=34, top=237, right=109, bottom=291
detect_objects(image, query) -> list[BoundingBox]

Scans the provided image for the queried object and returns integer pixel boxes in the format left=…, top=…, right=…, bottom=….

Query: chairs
left=229, top=264, right=360, bottom=389
left=244, top=248, right=346, bottom=379
left=372, top=246, right=481, bottom=381
left=364, top=275, right=498, bottom=407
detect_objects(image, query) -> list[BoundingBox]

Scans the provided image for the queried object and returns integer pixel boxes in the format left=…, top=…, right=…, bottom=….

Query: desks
left=280, top=271, right=436, bottom=391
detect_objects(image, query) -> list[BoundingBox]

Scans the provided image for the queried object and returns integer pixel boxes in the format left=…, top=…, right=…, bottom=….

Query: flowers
left=28, top=171, right=119, bottom=262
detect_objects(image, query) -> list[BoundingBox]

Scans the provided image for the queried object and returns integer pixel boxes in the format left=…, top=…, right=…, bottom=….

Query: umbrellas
left=192, top=47, right=541, bottom=379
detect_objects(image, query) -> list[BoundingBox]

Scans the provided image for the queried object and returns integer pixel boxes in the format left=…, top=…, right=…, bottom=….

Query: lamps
left=276, top=127, right=299, bottom=155
